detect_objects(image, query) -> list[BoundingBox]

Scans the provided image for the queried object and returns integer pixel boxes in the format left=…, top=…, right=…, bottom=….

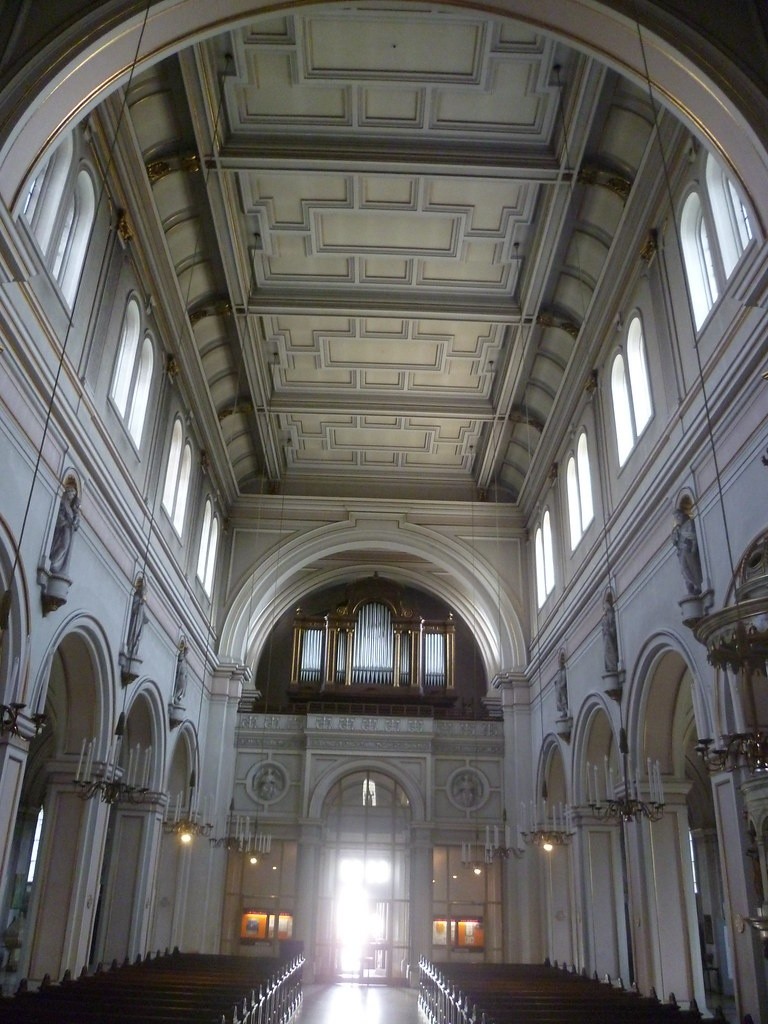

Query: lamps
left=516, top=241, right=565, bottom=849
left=209, top=352, right=279, bottom=852
left=552, top=62, right=666, bottom=826
left=160, top=230, right=261, bottom=843
left=488, top=360, right=528, bottom=863
left=242, top=439, right=291, bottom=862
left=74, top=49, right=234, bottom=806
left=634, top=17, right=768, bottom=680
left=0, top=0, right=151, bottom=742
left=651, top=227, right=768, bottom=778
left=460, top=444, right=493, bottom=875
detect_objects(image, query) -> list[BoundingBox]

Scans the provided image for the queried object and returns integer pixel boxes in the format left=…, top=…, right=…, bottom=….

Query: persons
left=174, top=647, right=190, bottom=705
left=602, top=594, right=619, bottom=672
left=460, top=775, right=475, bottom=807
left=260, top=769, right=276, bottom=799
left=554, top=651, right=568, bottom=719
left=128, top=577, right=150, bottom=658
left=49, top=485, right=78, bottom=573
left=671, top=510, right=703, bottom=595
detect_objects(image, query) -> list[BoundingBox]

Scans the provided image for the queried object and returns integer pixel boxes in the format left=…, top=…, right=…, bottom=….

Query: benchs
left=418, top=954, right=755, bottom=1024
left=0, top=945, right=307, bottom=1024
